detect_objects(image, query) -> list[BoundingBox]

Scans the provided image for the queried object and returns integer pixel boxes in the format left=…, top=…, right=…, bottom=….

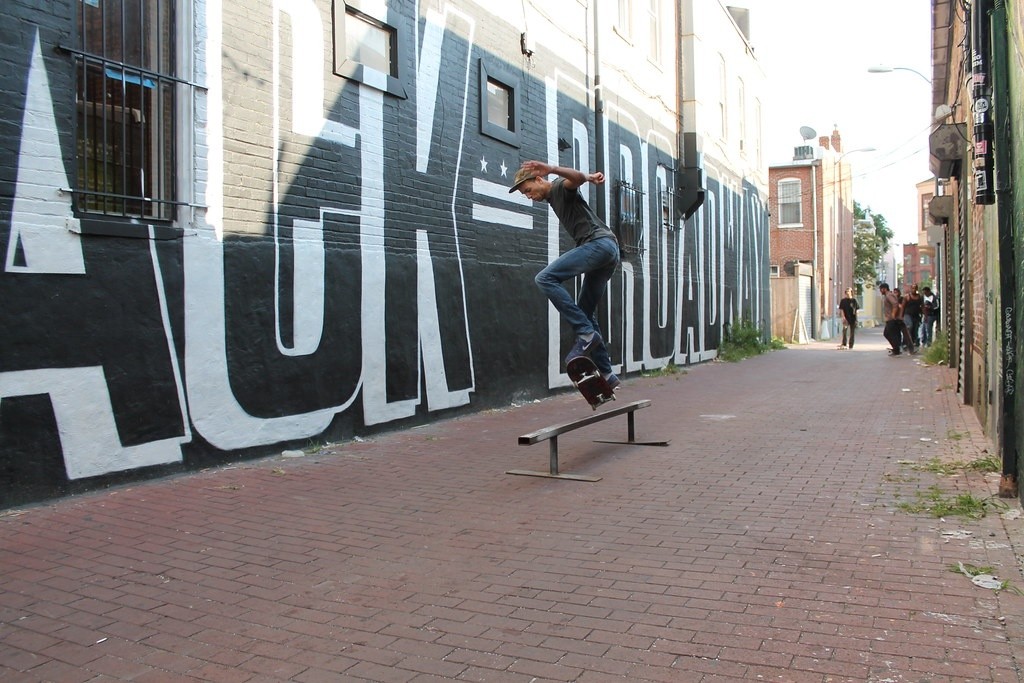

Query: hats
left=508, top=168, right=536, bottom=194
left=845, top=287, right=852, bottom=292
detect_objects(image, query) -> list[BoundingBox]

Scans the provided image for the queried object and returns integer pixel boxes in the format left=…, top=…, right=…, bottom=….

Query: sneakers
left=606, top=373, right=620, bottom=387
left=563, top=330, right=602, bottom=364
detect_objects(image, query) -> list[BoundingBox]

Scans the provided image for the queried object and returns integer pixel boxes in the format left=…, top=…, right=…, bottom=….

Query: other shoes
left=888, top=352, right=899, bottom=356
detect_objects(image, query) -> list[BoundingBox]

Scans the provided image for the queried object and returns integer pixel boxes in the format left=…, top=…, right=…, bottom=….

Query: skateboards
left=566, top=356, right=617, bottom=412
left=900, top=321, right=918, bottom=356
left=917, top=322, right=928, bottom=349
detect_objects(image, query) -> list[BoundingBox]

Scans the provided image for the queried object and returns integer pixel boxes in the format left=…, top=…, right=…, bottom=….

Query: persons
left=839, top=287, right=860, bottom=352
left=879, top=283, right=939, bottom=356
left=509, top=160, right=620, bottom=391
